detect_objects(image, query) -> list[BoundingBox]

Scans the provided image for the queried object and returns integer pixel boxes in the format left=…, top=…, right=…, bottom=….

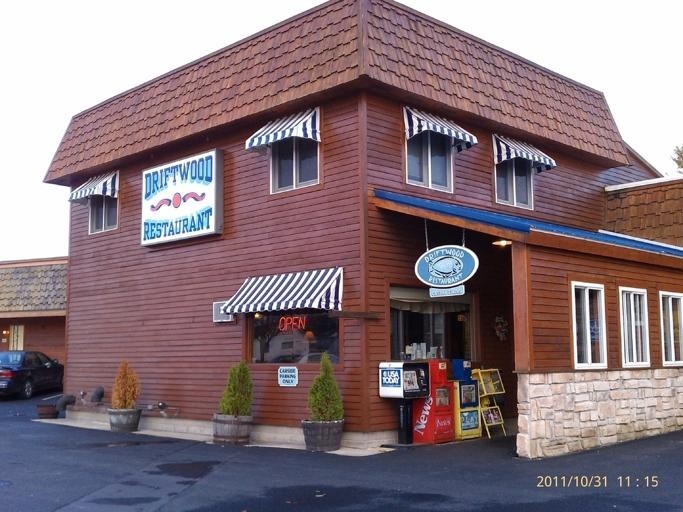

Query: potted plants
left=300, top=351, right=347, bottom=452
left=107, top=361, right=144, bottom=435
left=213, top=358, right=254, bottom=446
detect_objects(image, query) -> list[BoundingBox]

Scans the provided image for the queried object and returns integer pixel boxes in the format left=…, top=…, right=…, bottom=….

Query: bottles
left=416, top=343, right=423, bottom=360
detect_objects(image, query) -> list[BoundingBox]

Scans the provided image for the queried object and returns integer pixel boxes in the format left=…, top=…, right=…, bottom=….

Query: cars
left=0, top=350, right=64, bottom=399
left=264, top=351, right=337, bottom=364
